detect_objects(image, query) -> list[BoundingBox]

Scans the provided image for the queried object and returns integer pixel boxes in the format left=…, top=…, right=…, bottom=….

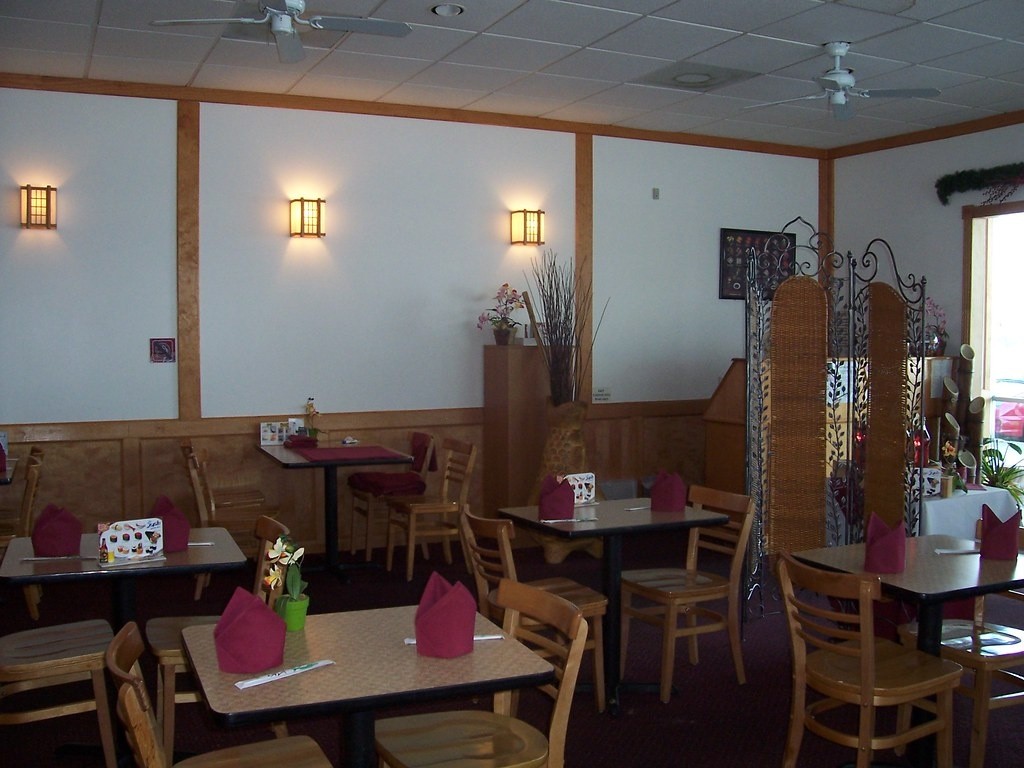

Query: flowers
left=476, top=282, right=525, bottom=331
left=263, top=533, right=309, bottom=600
left=930, top=441, right=968, bottom=494
left=305, top=397, right=322, bottom=428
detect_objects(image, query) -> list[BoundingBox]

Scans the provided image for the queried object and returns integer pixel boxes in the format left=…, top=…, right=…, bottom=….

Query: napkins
left=539, top=474, right=574, bottom=520
left=31, top=504, right=82, bottom=557
left=214, top=586, right=287, bottom=673
left=980, top=503, right=1022, bottom=559
left=650, top=468, right=687, bottom=512
left=151, top=495, right=190, bottom=553
left=415, top=571, right=476, bottom=659
left=864, top=511, right=907, bottom=573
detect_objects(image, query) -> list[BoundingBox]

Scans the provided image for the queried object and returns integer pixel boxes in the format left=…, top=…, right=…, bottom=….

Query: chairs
left=775, top=550, right=1024, bottom=767
left=0, top=431, right=755, bottom=768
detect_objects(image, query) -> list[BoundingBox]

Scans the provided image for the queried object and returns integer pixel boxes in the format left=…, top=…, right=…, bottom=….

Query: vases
left=275, top=595, right=309, bottom=631
left=494, top=328, right=518, bottom=345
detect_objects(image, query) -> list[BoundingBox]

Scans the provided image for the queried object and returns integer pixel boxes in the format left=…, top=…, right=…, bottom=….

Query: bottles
left=100, top=538, right=108, bottom=562
left=940, top=475, right=952, bottom=498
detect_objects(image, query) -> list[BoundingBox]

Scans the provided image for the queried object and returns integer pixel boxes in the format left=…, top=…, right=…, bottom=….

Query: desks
left=182, top=606, right=554, bottom=767
left=497, top=497, right=728, bottom=715
left=791, top=535, right=1024, bottom=768
left=0, top=528, right=247, bottom=768
left=258, top=441, right=414, bottom=585
left=906, top=486, right=1018, bottom=540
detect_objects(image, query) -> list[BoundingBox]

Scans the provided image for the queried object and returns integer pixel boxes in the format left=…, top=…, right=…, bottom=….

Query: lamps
left=20, top=185, right=545, bottom=247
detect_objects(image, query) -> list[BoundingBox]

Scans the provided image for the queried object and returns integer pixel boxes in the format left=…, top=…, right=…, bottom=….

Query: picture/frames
left=719, top=228, right=797, bottom=301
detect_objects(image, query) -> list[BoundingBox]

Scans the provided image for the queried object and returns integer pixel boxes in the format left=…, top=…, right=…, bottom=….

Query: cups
left=108, top=551, right=114, bottom=562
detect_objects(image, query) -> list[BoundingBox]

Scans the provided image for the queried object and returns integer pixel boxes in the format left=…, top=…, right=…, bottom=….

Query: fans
left=743, top=42, right=942, bottom=123
left=149, top=0, right=413, bottom=64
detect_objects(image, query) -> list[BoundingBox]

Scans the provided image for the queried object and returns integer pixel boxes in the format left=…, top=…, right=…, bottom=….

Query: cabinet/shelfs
left=298, top=427, right=319, bottom=438
